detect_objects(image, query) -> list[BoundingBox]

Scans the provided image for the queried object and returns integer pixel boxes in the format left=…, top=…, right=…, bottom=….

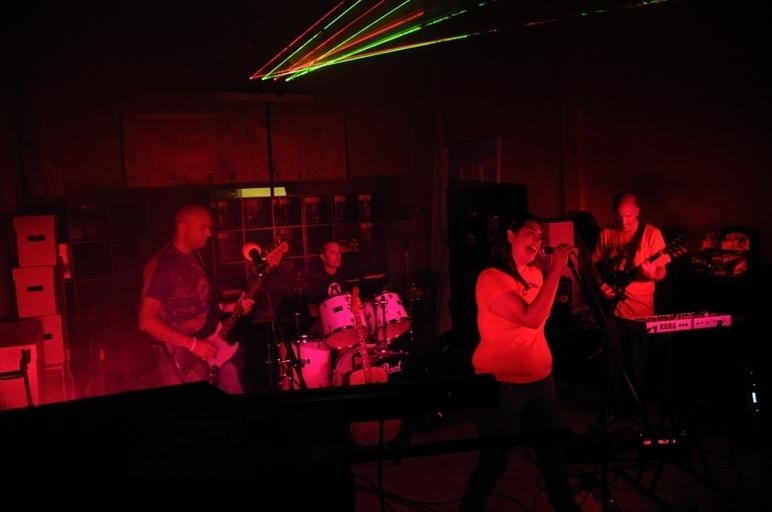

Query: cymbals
left=346, top=274, right=386, bottom=283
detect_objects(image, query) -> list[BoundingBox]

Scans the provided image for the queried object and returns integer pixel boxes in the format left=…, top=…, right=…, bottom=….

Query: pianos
left=630, top=309, right=734, bottom=335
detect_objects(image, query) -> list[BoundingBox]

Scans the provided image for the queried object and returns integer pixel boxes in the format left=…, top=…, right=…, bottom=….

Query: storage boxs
left=0, top=215, right=78, bottom=411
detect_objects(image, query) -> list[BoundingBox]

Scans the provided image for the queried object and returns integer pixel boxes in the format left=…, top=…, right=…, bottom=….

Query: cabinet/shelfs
left=64, top=176, right=391, bottom=325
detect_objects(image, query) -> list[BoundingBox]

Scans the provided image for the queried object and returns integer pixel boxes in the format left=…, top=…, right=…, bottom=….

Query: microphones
left=241, top=241, right=267, bottom=273
left=538, top=244, right=558, bottom=257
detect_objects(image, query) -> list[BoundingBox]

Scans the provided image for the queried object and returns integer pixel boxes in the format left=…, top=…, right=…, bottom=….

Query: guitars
left=581, top=239, right=689, bottom=314
left=344, top=286, right=389, bottom=385
left=173, top=240, right=289, bottom=384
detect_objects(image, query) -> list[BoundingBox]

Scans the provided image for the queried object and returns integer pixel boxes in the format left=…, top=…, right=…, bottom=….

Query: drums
left=333, top=344, right=413, bottom=388
left=363, top=292, right=412, bottom=344
left=267, top=335, right=334, bottom=391
left=318, top=292, right=369, bottom=350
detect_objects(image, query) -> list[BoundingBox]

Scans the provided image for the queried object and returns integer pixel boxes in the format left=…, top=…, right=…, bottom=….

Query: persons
left=695, top=228, right=750, bottom=276
left=135, top=205, right=256, bottom=396
left=459, top=211, right=581, bottom=511
left=303, top=240, right=353, bottom=341
left=587, top=191, right=674, bottom=427
